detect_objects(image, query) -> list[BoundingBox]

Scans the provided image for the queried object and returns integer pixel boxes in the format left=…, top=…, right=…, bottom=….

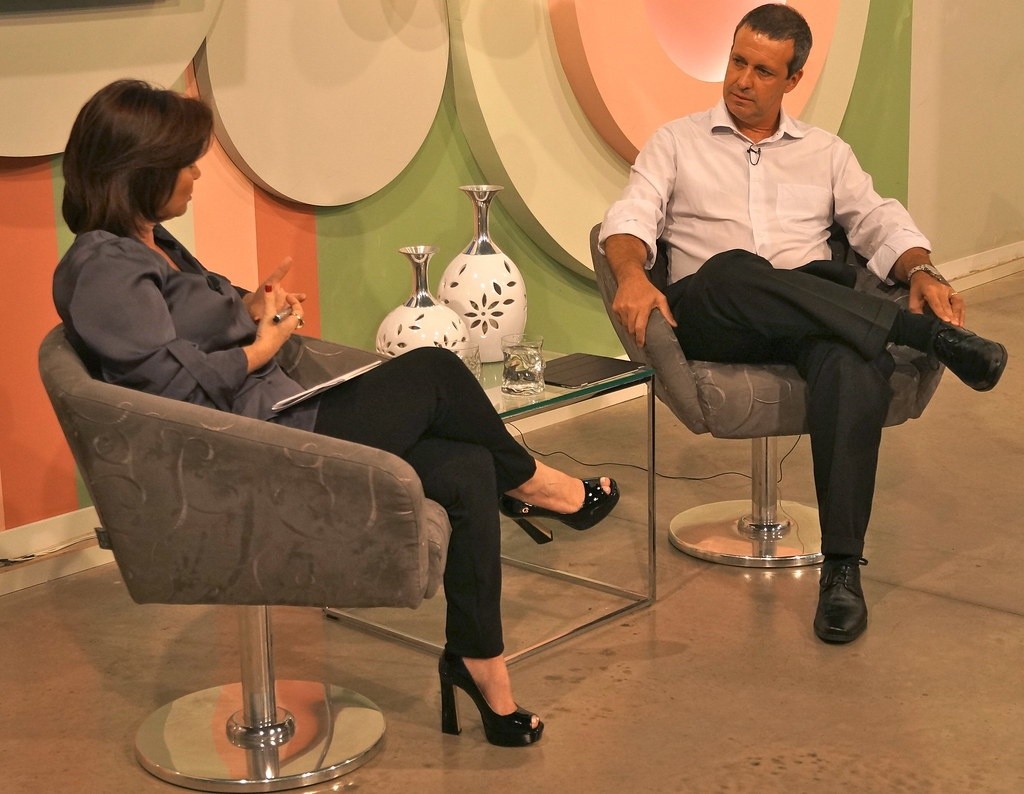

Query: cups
left=500, top=333, right=546, bottom=396
left=436, top=340, right=480, bottom=381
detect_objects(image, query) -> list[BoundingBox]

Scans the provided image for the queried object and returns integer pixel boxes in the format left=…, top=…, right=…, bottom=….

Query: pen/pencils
left=274, top=307, right=293, bottom=322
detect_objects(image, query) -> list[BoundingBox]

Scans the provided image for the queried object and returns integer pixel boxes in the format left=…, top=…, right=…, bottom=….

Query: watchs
left=908, top=264, right=950, bottom=284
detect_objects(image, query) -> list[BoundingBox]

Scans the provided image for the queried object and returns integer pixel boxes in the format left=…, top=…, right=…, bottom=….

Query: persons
left=597, top=3, right=1008, bottom=643
left=52, top=78, right=622, bottom=748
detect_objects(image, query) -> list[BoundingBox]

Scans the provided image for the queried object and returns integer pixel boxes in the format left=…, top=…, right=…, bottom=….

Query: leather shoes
left=813, top=556, right=869, bottom=644
left=925, top=316, right=1009, bottom=393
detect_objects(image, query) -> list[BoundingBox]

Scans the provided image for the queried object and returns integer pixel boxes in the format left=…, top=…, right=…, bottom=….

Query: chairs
left=34, top=292, right=466, bottom=788
left=581, top=213, right=956, bottom=563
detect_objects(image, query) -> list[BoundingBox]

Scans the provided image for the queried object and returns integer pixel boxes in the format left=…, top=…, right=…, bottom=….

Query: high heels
left=498, top=473, right=621, bottom=545
left=437, top=642, right=545, bottom=748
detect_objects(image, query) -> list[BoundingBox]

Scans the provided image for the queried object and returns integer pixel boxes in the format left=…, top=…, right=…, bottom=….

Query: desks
left=325, top=329, right=664, bottom=675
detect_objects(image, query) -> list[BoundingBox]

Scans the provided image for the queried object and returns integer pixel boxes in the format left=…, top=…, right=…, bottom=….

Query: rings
left=289, top=312, right=306, bottom=330
